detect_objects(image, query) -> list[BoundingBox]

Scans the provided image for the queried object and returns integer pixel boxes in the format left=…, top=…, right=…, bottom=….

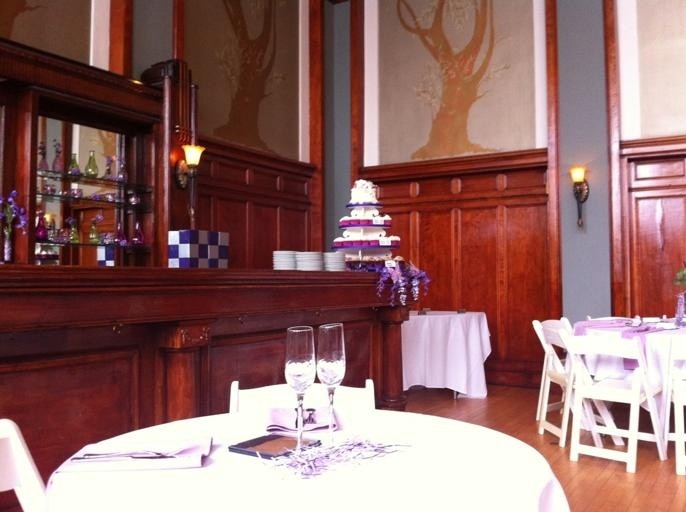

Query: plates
left=269, top=250, right=344, bottom=273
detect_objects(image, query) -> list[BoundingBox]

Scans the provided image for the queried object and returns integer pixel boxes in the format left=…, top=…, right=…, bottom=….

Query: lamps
left=569, top=167, right=590, bottom=227
left=173, top=144, right=206, bottom=210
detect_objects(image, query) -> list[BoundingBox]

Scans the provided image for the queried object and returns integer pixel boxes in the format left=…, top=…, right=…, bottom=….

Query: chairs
left=0, top=418, right=47, bottom=512
left=227, top=377, right=375, bottom=415
left=566, top=328, right=668, bottom=475
left=671, top=377, right=686, bottom=478
left=531, top=316, right=627, bottom=449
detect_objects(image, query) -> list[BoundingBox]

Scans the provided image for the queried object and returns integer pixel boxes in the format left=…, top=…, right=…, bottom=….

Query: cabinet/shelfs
left=0, top=327, right=155, bottom=512
left=31, top=169, right=155, bottom=267
left=204, top=319, right=378, bottom=415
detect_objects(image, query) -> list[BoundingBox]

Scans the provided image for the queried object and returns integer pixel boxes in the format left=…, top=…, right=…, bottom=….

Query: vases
left=38, top=158, right=48, bottom=170
left=51, top=156, right=63, bottom=172
left=1, top=229, right=14, bottom=263
left=105, top=166, right=111, bottom=176
left=68, top=225, right=79, bottom=243
left=32, top=212, right=47, bottom=241
left=117, top=168, right=128, bottom=182
left=88, top=223, right=98, bottom=244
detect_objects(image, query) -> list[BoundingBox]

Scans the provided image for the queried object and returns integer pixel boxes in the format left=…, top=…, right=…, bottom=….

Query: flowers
left=51, top=139, right=62, bottom=157
left=116, top=156, right=126, bottom=167
left=63, top=216, right=77, bottom=225
left=0, top=188, right=29, bottom=236
left=36, top=141, right=47, bottom=159
left=90, top=215, right=104, bottom=224
left=344, top=257, right=431, bottom=308
left=103, top=155, right=112, bottom=166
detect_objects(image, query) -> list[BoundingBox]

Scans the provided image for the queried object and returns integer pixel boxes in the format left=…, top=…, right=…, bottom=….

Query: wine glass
left=281, top=321, right=346, bottom=442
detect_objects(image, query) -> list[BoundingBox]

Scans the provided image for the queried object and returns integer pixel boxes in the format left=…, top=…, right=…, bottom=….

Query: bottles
left=84, top=150, right=99, bottom=178
left=69, top=153, right=78, bottom=169
